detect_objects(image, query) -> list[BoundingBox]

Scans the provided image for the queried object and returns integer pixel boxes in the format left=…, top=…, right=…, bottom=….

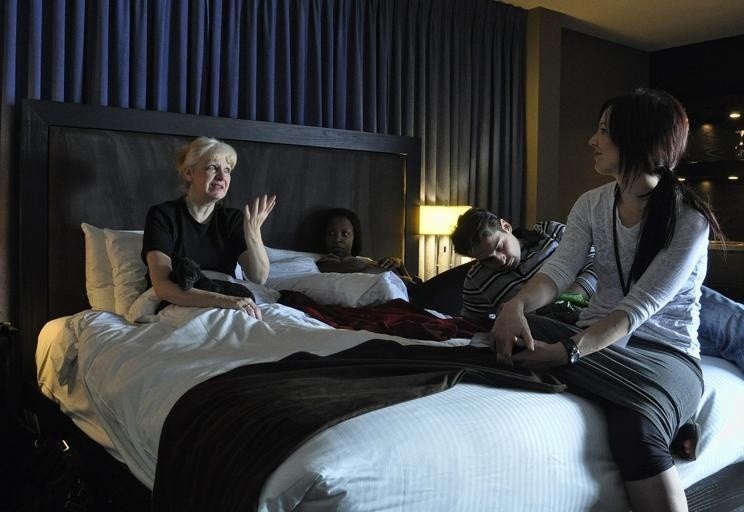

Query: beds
left=18, top=100, right=744, bottom=511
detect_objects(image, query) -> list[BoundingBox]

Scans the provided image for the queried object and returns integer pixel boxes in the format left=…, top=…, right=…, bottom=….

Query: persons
left=142, top=136, right=278, bottom=319
left=452, top=202, right=743, bottom=371
left=491, top=92, right=710, bottom=512
left=316, top=207, right=479, bottom=315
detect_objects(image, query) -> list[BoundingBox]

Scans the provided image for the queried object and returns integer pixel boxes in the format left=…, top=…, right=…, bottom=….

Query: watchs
left=560, top=337, right=579, bottom=365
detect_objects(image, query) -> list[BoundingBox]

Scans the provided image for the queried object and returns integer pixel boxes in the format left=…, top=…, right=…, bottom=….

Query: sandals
left=677, top=414, right=702, bottom=460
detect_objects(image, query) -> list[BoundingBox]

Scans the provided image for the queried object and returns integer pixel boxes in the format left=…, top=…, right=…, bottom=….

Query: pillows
left=264, top=246, right=322, bottom=262
left=105, top=228, right=146, bottom=317
left=81, top=223, right=116, bottom=314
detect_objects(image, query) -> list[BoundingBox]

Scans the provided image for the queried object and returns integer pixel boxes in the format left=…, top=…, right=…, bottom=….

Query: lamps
left=419, top=205, right=472, bottom=275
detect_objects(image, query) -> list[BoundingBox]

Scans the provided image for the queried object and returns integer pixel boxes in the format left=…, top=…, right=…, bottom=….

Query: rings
left=243, top=305, right=251, bottom=309
